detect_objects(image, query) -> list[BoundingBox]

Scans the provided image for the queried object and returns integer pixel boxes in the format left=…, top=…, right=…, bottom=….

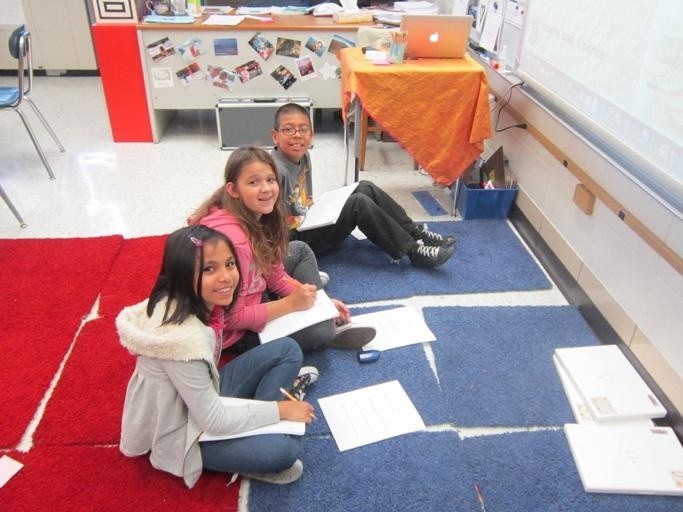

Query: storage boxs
left=462, top=137, right=505, bottom=183
left=452, top=170, right=517, bottom=218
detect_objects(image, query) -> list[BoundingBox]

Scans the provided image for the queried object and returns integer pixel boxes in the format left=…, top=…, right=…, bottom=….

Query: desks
left=137, top=5, right=384, bottom=146
left=340, top=46, right=482, bottom=182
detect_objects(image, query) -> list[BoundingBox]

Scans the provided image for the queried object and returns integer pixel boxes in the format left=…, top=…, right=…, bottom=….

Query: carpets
left=0, top=218, right=682, bottom=511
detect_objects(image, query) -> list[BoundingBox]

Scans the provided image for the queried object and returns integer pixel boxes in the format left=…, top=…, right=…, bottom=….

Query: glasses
left=276, top=126, right=312, bottom=135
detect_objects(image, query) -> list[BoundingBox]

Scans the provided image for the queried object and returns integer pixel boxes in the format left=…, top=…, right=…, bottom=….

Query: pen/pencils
left=389, top=31, right=409, bottom=43
left=279, top=387, right=317, bottom=420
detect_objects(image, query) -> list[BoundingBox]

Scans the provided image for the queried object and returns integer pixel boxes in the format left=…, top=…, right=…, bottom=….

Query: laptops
left=399, top=13, right=475, bottom=59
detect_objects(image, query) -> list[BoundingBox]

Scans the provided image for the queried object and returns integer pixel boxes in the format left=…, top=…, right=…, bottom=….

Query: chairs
left=0, top=24, right=66, bottom=181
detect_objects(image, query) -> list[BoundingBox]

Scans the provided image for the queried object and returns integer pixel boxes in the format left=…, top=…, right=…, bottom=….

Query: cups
left=144, top=0, right=206, bottom=18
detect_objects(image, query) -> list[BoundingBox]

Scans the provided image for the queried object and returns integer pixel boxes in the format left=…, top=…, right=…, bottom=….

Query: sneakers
left=327, top=325, right=376, bottom=351
left=318, top=272, right=329, bottom=285
left=409, top=223, right=456, bottom=268
left=234, top=458, right=303, bottom=485
left=283, top=366, right=319, bottom=401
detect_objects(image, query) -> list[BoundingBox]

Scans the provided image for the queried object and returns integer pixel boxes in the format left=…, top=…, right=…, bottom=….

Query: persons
left=158, top=32, right=323, bottom=90
left=267, top=101, right=457, bottom=270
left=113, top=222, right=319, bottom=491
left=185, top=145, right=377, bottom=355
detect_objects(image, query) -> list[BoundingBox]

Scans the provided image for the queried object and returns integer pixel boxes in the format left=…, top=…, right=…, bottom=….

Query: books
left=257, top=286, right=339, bottom=346
left=553, top=344, right=676, bottom=422
left=563, top=426, right=682, bottom=496
left=550, top=353, right=654, bottom=425
left=197, top=421, right=305, bottom=442
left=295, top=180, right=360, bottom=233
left=393, top=0, right=440, bottom=14
